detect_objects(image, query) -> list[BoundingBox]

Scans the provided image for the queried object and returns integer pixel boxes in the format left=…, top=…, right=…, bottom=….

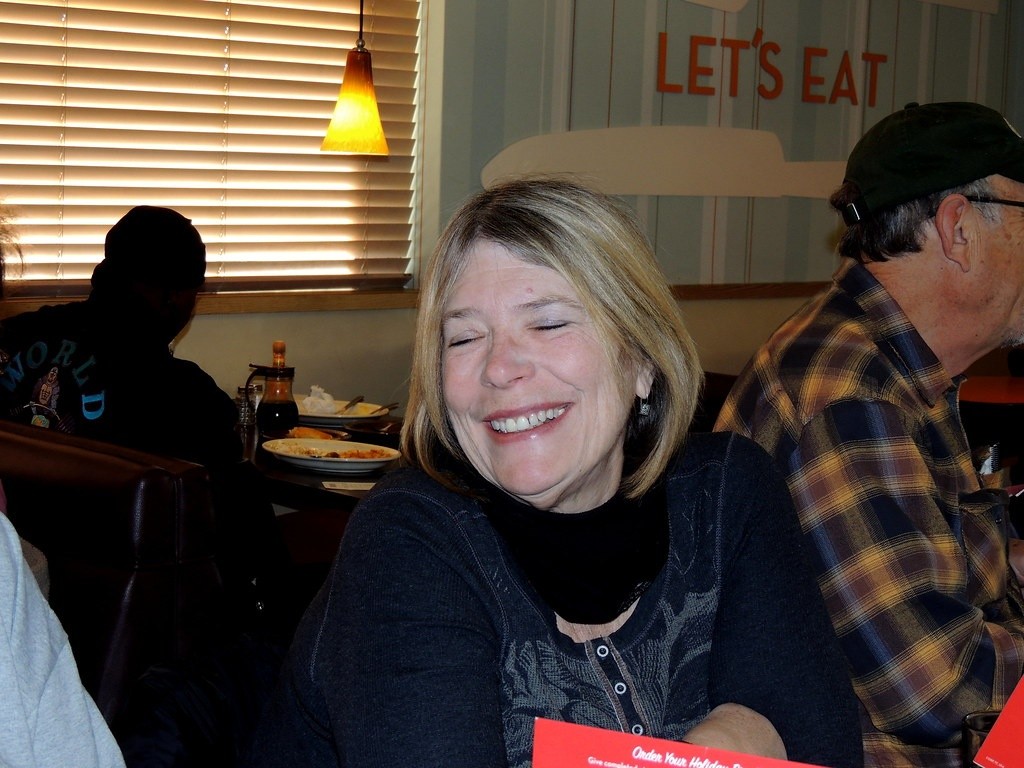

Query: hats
left=841, top=102, right=1024, bottom=223
left=104, top=206, right=206, bottom=294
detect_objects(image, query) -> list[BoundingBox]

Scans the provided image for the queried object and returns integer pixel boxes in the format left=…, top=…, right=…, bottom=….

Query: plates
left=295, top=399, right=389, bottom=427
left=288, top=428, right=348, bottom=440
left=262, top=438, right=402, bottom=473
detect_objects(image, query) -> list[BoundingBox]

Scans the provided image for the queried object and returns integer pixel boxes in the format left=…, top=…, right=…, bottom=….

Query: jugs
left=245, top=341, right=298, bottom=454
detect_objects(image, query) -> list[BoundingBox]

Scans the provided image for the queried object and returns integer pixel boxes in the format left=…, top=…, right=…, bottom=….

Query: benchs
left=0, top=419, right=246, bottom=768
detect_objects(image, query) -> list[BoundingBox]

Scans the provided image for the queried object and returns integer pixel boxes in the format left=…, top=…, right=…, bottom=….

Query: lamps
left=322, top=0, right=390, bottom=155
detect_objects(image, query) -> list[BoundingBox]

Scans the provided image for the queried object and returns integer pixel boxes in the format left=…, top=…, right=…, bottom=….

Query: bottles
left=248, top=382, right=265, bottom=465
left=234, top=386, right=250, bottom=461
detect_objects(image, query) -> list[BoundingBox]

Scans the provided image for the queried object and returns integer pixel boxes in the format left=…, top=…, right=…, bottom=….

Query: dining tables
left=228, top=397, right=408, bottom=515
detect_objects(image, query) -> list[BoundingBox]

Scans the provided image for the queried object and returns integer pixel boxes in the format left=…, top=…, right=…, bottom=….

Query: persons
left=247, top=175, right=865, bottom=768
left=0, top=512, right=125, bottom=768
left=0, top=204, right=294, bottom=657
left=713, top=102, right=1024, bottom=768
